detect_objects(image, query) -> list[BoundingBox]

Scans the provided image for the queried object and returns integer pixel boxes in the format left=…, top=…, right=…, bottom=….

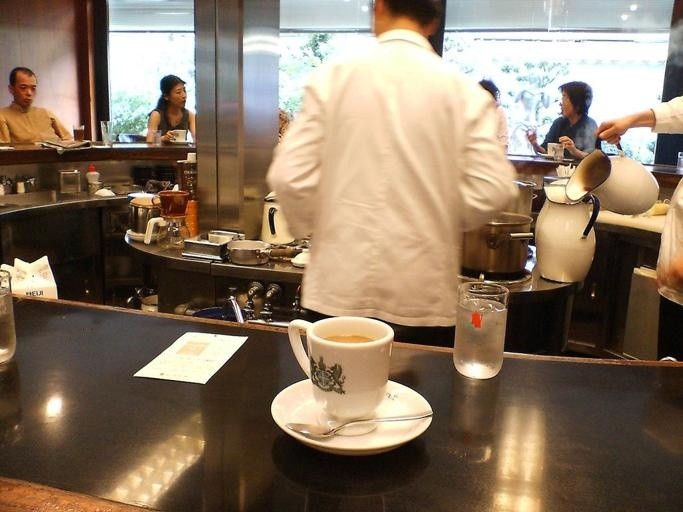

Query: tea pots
left=143, top=217, right=192, bottom=250
left=534, top=177, right=600, bottom=283
left=565, top=133, right=660, bottom=215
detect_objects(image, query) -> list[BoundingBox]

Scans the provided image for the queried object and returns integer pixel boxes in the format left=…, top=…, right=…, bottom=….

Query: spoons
left=285, top=410, right=433, bottom=438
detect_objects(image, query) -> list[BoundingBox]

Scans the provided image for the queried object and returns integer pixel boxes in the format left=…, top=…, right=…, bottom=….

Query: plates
left=542, top=154, right=554, bottom=158
left=126, top=230, right=157, bottom=243
left=170, top=140, right=191, bottom=145
left=271, top=432, right=431, bottom=497
left=270, top=378, right=433, bottom=455
left=126, top=228, right=162, bottom=244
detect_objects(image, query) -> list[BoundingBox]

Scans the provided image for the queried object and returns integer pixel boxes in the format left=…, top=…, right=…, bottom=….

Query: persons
left=0, top=67, right=72, bottom=149
left=526, top=82, right=598, bottom=160
left=145, top=75, right=196, bottom=143
left=478, top=80, right=508, bottom=153
left=264, top=1, right=519, bottom=348
left=594, top=96, right=683, bottom=361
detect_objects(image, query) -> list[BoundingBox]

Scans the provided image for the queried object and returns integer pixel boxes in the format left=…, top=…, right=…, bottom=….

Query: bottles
left=0, top=176, right=37, bottom=196
left=86, top=164, right=99, bottom=182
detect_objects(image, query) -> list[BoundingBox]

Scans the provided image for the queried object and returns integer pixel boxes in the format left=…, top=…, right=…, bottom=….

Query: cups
left=152, top=130, right=162, bottom=145
left=452, top=281, right=510, bottom=379
left=287, top=315, right=395, bottom=414
left=304, top=489, right=385, bottom=511
left=0, top=359, right=24, bottom=447
left=73, top=124, right=84, bottom=142
left=548, top=143, right=564, bottom=154
left=172, top=130, right=186, bottom=142
left=676, top=152, right=683, bottom=170
left=0, top=269, right=16, bottom=365
left=446, top=369, right=499, bottom=464
left=100, top=121, right=113, bottom=144
left=151, top=190, right=190, bottom=217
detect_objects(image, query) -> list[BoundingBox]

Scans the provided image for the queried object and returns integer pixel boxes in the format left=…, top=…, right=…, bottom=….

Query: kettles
left=260, top=191, right=296, bottom=245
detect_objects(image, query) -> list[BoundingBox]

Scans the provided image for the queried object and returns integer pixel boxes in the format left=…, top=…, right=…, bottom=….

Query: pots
left=227, top=240, right=302, bottom=265
left=505, top=180, right=537, bottom=216
left=464, top=214, right=533, bottom=274
